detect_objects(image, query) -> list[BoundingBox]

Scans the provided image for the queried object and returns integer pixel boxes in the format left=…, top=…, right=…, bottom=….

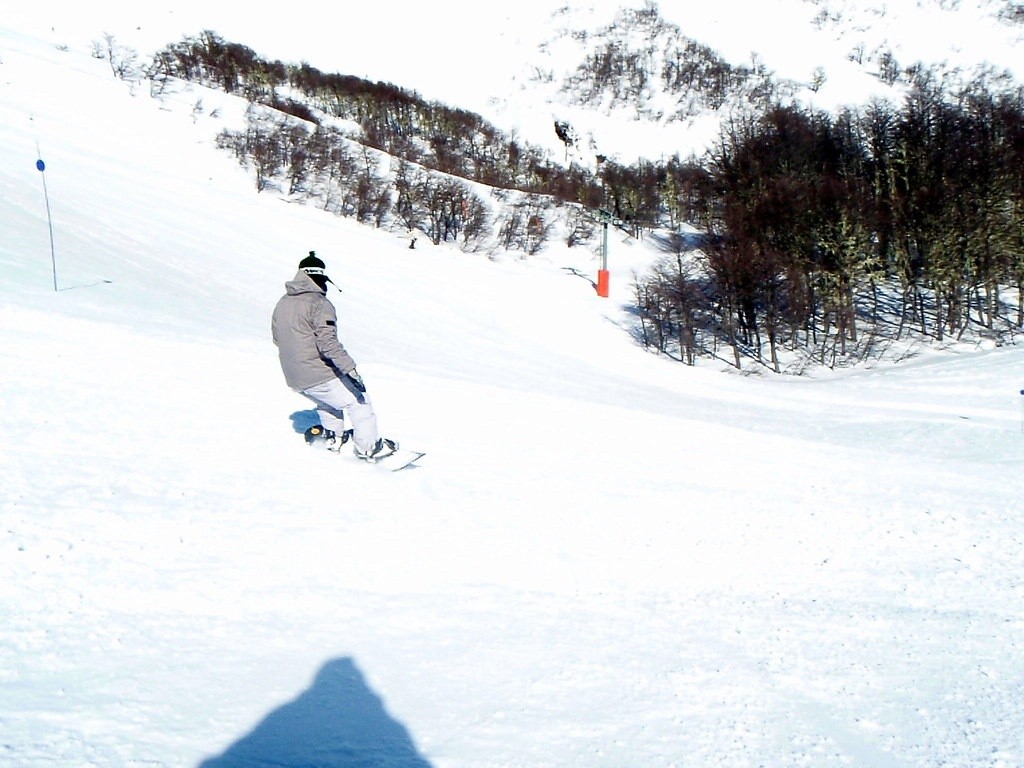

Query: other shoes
left=313, top=434, right=342, bottom=452
left=356, top=441, right=395, bottom=458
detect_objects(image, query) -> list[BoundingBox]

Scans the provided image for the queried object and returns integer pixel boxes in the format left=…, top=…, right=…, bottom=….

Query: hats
left=299, top=251, right=325, bottom=274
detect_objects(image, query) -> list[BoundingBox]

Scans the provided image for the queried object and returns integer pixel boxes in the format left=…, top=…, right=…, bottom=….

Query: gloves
left=345, top=369, right=366, bottom=392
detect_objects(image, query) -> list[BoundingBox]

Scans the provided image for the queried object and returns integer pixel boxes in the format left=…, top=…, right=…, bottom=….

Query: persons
left=271, top=251, right=397, bottom=458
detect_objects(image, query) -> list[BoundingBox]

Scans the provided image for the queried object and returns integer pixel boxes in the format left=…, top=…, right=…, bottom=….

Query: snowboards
left=304, top=424, right=425, bottom=472
